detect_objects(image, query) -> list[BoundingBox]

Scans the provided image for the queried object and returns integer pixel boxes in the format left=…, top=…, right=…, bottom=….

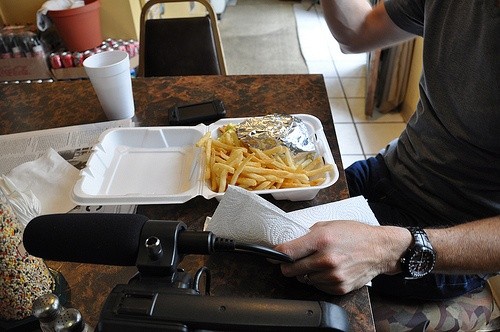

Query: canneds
left=48, top=37, right=138, bottom=69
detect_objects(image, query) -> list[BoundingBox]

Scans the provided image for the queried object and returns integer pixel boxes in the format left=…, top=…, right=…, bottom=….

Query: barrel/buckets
left=47, top=0, right=102, bottom=52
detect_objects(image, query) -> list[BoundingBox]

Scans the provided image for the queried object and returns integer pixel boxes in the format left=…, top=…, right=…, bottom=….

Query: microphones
left=23, top=212, right=234, bottom=266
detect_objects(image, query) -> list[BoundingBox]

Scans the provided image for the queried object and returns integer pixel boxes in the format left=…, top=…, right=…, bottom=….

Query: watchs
left=397, top=225, right=438, bottom=283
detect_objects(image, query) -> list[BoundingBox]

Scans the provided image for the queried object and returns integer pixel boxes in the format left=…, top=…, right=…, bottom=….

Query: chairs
left=138, top=0, right=227, bottom=76
left=373, top=273, right=500, bottom=332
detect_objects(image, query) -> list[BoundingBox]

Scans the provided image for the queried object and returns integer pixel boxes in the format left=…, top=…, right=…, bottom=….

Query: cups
left=83, top=50, right=135, bottom=121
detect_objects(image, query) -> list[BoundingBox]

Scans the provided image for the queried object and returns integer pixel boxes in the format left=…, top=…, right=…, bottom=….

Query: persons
left=273, top=0, right=500, bottom=295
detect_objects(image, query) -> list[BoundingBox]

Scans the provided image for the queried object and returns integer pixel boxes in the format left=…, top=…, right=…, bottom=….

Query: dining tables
left=0, top=73, right=378, bottom=332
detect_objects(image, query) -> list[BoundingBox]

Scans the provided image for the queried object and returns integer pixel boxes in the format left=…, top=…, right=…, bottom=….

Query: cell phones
left=168, top=99, right=226, bottom=126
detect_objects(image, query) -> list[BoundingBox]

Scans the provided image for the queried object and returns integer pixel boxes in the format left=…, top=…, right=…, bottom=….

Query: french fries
left=195, top=124, right=334, bottom=194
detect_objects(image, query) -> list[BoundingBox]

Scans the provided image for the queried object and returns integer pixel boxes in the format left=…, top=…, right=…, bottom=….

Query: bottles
left=0, top=23, right=60, bottom=58
left=33, top=294, right=67, bottom=332
left=54, top=308, right=94, bottom=332
